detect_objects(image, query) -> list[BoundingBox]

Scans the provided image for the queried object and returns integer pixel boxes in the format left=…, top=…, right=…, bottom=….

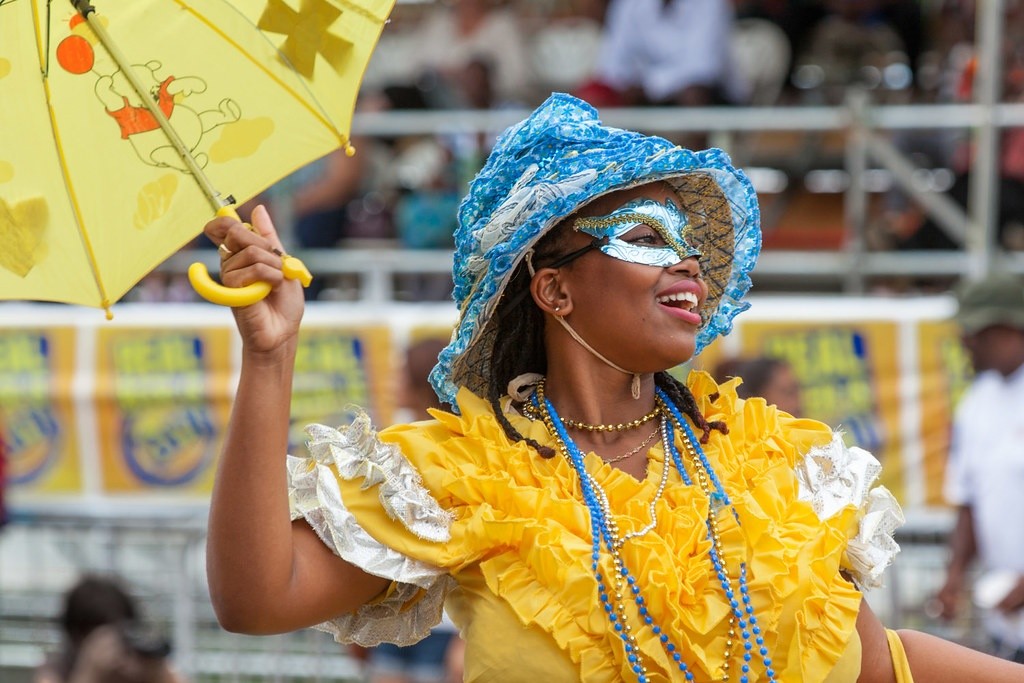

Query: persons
left=346, top=333, right=473, bottom=683
left=717, top=352, right=806, bottom=419
left=924, top=274, right=1021, bottom=662
left=200, top=86, right=1024, bottom=682
left=39, top=577, right=186, bottom=683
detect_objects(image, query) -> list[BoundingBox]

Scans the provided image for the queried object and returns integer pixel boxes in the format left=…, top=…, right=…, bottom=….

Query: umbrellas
left=0, top=0, right=403, bottom=319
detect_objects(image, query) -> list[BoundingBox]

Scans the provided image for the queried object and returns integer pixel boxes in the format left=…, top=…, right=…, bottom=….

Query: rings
left=217, top=244, right=232, bottom=259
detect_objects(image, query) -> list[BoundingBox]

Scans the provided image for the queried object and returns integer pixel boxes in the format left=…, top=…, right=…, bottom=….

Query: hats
left=425, top=90, right=763, bottom=417
left=940, top=271, right=1024, bottom=339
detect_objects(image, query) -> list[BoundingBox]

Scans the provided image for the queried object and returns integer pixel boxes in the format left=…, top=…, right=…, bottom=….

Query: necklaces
left=521, top=378, right=779, bottom=683
